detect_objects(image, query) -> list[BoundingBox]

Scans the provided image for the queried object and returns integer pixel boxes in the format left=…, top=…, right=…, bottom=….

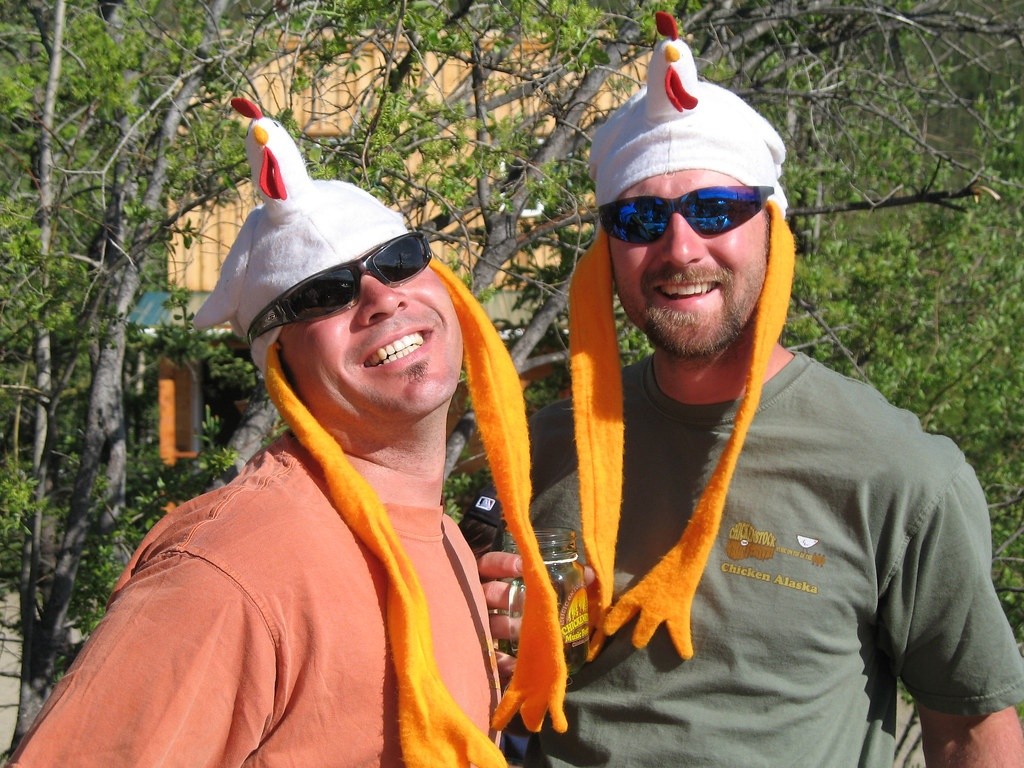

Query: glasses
left=597, top=188, right=776, bottom=244
left=247, top=233, right=432, bottom=347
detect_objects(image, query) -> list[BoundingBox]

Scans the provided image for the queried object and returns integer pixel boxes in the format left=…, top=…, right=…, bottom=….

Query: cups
left=493, top=527, right=590, bottom=678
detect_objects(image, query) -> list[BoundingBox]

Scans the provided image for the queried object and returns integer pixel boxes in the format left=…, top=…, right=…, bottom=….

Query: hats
left=565, top=12, right=795, bottom=664
left=192, top=100, right=570, bottom=768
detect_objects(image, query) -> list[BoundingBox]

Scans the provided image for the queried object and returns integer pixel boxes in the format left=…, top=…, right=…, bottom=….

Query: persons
left=2, top=178, right=509, bottom=767
left=466, top=84, right=1018, bottom=767
